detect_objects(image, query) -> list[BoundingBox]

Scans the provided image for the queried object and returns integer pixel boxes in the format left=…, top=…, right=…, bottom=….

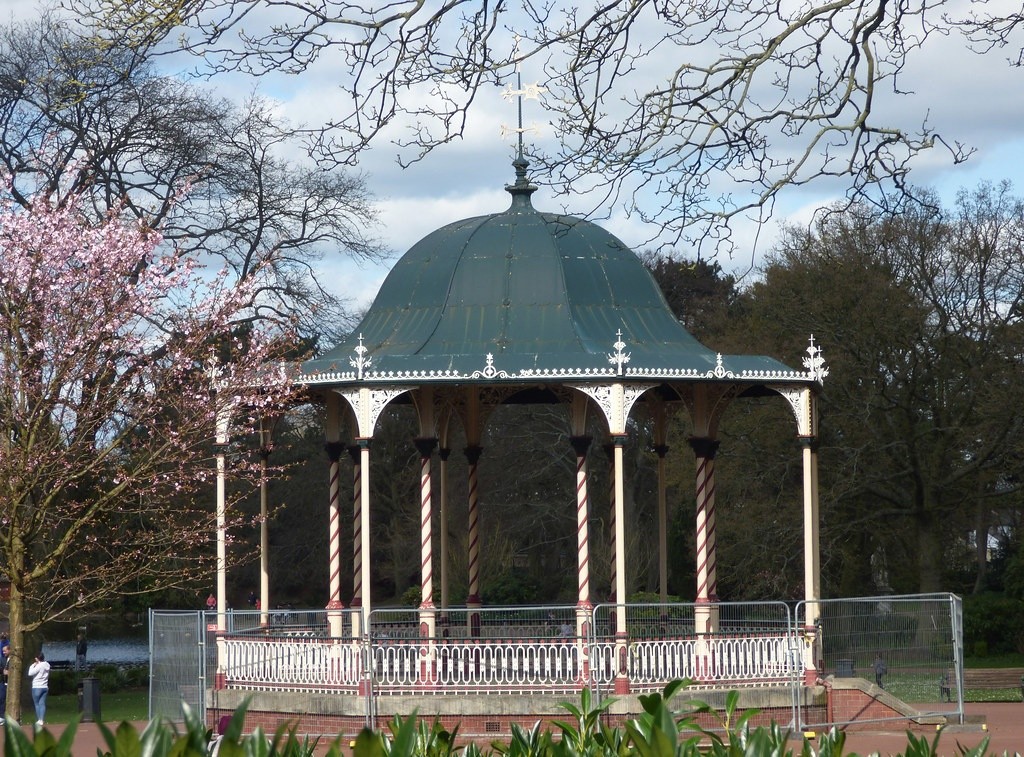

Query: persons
left=206, top=593, right=216, bottom=609
left=870, top=651, right=888, bottom=687
left=248, top=591, right=261, bottom=621
left=28, top=653, right=50, bottom=725
left=2, top=645, right=10, bottom=686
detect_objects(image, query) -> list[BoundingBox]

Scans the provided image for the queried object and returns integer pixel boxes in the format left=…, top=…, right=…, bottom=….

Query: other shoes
left=0, top=721, right=5, bottom=726
left=35, top=719, right=44, bottom=726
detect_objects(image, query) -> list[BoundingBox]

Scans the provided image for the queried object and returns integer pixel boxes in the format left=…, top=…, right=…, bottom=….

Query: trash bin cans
left=80, top=678, right=101, bottom=722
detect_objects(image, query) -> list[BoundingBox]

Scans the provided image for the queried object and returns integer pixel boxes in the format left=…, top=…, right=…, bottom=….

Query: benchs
left=47, top=661, right=70, bottom=672
